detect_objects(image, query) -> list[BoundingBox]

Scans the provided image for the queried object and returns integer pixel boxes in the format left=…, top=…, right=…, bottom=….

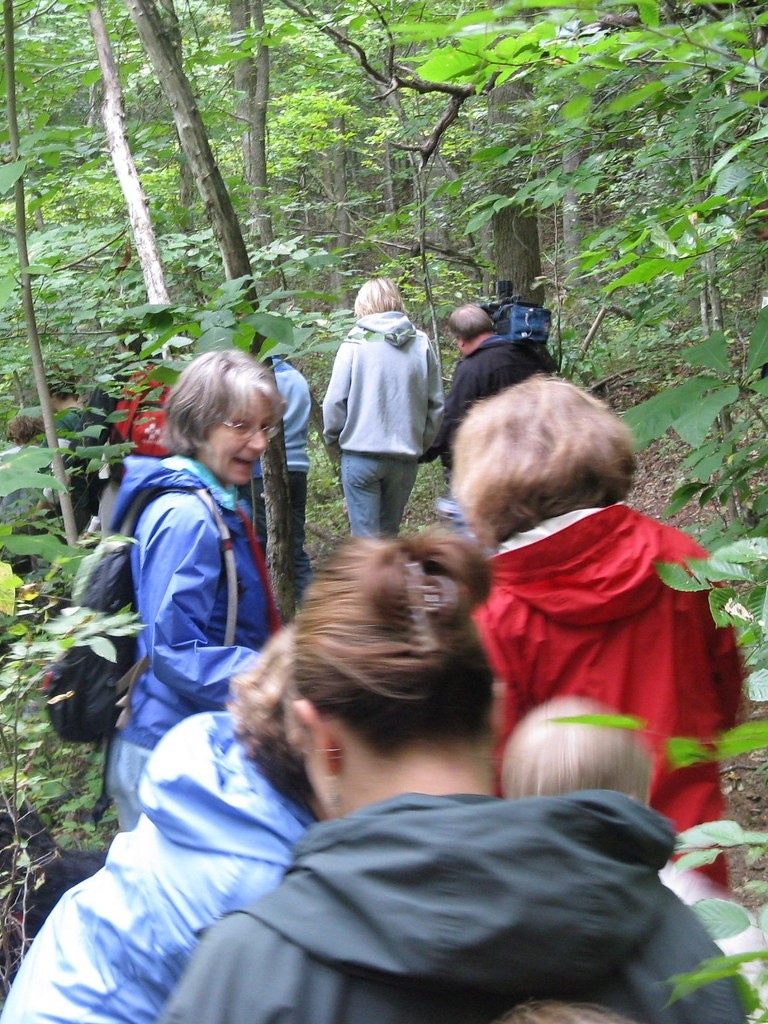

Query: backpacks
left=111, top=362, right=171, bottom=456
left=490, top=303, right=553, bottom=343
left=48, top=486, right=226, bottom=742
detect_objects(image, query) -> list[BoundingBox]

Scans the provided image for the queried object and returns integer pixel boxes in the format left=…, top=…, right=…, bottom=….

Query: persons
left=0, top=624, right=324, bottom=1024
left=157, top=530, right=748, bottom=1024
left=100, top=347, right=283, bottom=830
left=434, top=307, right=560, bottom=543
left=449, top=375, right=748, bottom=902
left=321, top=278, right=447, bottom=544
left=499, top=694, right=767, bottom=1023
left=1, top=322, right=172, bottom=598
left=231, top=312, right=314, bottom=609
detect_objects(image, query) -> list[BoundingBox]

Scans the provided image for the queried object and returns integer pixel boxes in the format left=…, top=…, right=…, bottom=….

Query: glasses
left=222, top=419, right=281, bottom=439
